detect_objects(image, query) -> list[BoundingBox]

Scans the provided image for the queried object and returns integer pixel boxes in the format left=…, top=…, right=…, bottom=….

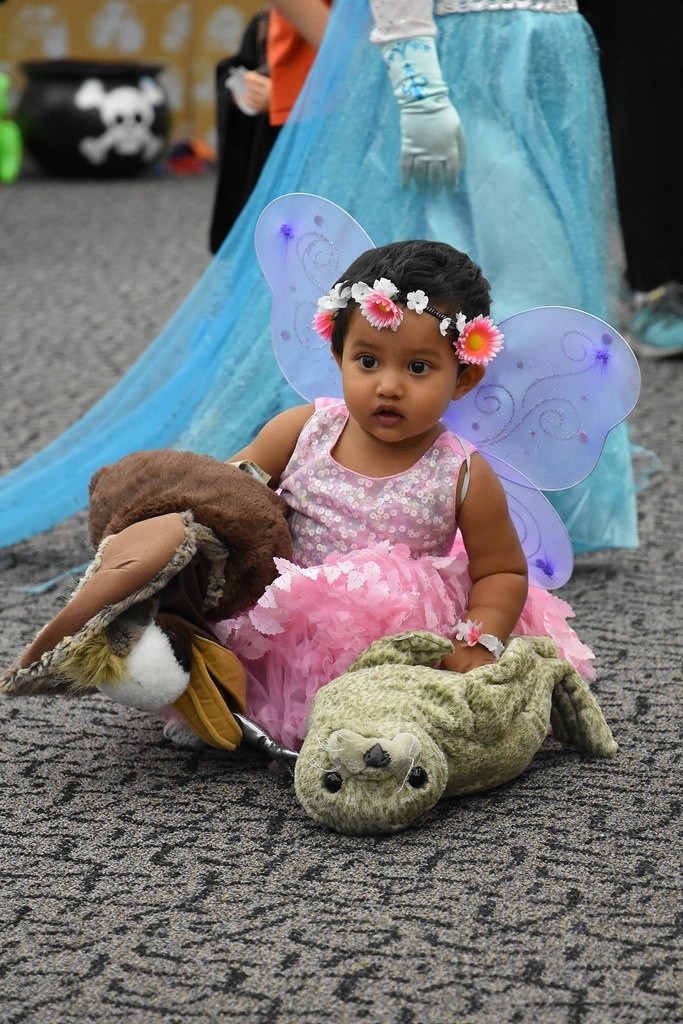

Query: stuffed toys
left=0, top=449, right=294, bottom=753
left=294, top=629, right=618, bottom=835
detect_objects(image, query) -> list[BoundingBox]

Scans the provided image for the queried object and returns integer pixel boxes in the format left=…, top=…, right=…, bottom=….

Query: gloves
left=380, top=37, right=463, bottom=193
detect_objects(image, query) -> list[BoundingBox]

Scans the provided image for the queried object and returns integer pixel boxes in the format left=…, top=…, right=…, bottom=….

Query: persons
left=208, top=0, right=683, bottom=557
left=162, top=239, right=598, bottom=756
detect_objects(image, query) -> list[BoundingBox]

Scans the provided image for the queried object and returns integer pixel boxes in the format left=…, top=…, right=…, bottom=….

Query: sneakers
left=622, top=280, right=683, bottom=360
